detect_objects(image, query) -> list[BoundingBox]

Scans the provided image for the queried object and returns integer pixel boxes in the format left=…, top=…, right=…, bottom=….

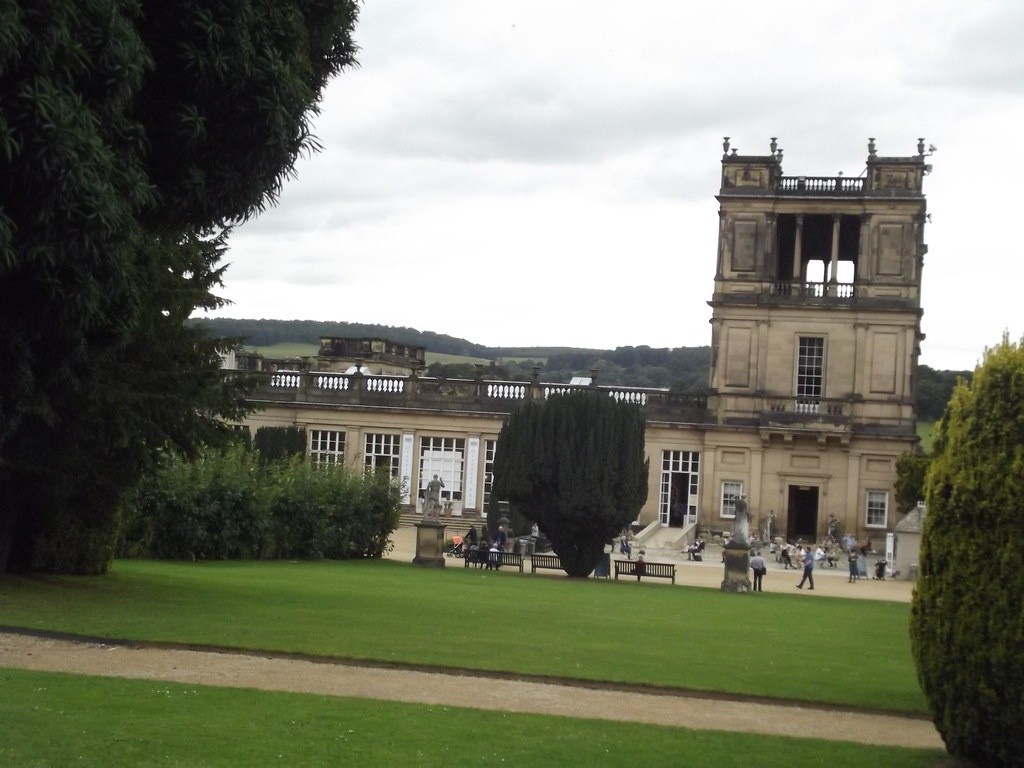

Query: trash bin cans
left=909, top=565, right=918, bottom=581
left=528, top=538, right=536, bottom=554
left=519, top=539, right=528, bottom=556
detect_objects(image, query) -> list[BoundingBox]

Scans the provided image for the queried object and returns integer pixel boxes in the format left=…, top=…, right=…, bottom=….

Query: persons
left=426, top=474, right=445, bottom=521
left=767, top=509, right=777, bottom=534
left=620, top=528, right=639, bottom=560
left=463, top=522, right=538, bottom=569
left=730, top=493, right=751, bottom=549
left=750, top=551, right=764, bottom=592
left=635, top=554, right=645, bottom=581
left=687, top=535, right=705, bottom=561
left=827, top=512, right=840, bottom=543
left=795, top=546, right=815, bottom=590
left=720, top=534, right=872, bottom=570
left=847, top=548, right=858, bottom=583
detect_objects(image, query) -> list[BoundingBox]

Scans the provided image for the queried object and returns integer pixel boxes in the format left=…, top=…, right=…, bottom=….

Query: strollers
left=447, top=535, right=466, bottom=558
left=873, top=559, right=888, bottom=580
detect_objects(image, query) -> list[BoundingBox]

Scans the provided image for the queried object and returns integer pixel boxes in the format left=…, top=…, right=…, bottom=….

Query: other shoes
left=797, top=586, right=802, bottom=588
left=808, top=588, right=814, bottom=589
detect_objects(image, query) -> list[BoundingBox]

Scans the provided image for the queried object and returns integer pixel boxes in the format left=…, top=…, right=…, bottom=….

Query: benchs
left=463, top=549, right=523, bottom=573
left=614, top=560, right=677, bottom=585
left=531, top=554, right=564, bottom=575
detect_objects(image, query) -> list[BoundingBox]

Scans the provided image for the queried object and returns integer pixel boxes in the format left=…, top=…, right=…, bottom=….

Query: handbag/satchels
left=762, top=567, right=766, bottom=575
